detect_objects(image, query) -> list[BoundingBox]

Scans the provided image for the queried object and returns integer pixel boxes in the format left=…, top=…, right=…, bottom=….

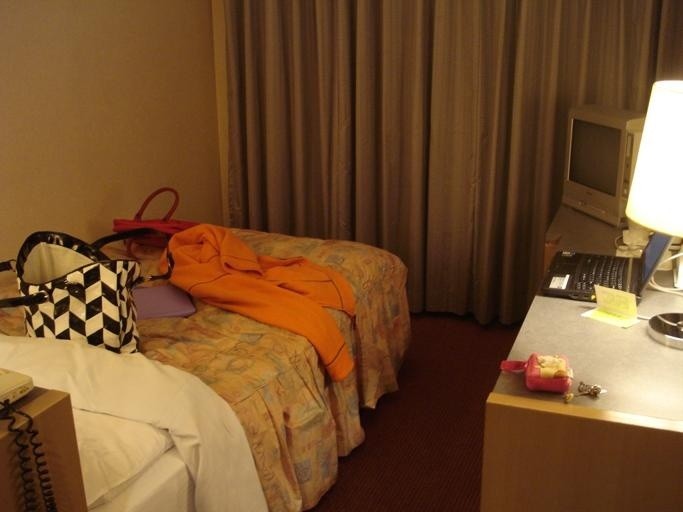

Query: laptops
left=542, top=230, right=673, bottom=306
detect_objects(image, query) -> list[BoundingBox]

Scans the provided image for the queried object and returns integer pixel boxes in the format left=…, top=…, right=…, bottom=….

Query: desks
left=478, top=251, right=683, bottom=512
left=537, top=205, right=620, bottom=283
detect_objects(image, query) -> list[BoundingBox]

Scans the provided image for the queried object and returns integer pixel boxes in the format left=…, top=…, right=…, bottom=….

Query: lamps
left=625, top=80, right=683, bottom=350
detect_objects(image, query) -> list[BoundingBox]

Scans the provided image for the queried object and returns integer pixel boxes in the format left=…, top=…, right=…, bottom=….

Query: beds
left=0, top=224, right=413, bottom=512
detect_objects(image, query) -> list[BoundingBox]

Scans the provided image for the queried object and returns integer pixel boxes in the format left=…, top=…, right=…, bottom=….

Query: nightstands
left=1, top=386, right=89, bottom=512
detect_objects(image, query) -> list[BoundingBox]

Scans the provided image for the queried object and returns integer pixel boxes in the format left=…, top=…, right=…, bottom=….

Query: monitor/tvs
left=561, top=107, right=645, bottom=229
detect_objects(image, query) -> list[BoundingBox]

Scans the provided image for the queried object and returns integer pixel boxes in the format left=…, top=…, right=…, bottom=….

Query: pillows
left=72, top=405, right=174, bottom=512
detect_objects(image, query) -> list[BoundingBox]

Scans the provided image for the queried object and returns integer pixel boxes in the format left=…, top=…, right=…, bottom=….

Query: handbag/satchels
left=112, top=218, right=200, bottom=248
left=13, top=232, right=139, bottom=355
left=128, top=285, right=195, bottom=321
left=524, top=353, right=573, bottom=394
